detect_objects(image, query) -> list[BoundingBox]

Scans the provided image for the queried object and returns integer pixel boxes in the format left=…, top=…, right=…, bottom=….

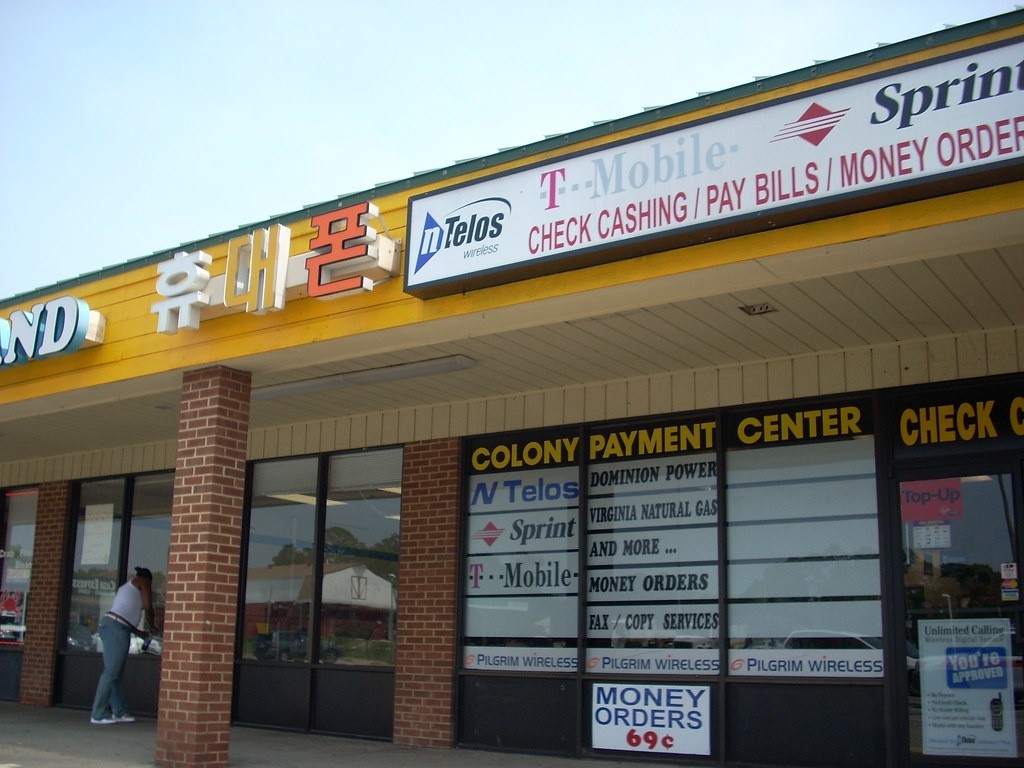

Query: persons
left=90, top=567, right=159, bottom=724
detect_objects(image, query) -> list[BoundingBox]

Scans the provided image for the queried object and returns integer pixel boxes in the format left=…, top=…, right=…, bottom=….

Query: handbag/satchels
left=141, top=635, right=164, bottom=656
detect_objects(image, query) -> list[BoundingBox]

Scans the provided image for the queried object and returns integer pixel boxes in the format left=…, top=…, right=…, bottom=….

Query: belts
left=104, top=614, right=129, bottom=626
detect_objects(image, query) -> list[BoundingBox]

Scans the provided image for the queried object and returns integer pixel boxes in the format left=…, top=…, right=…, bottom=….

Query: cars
left=916, top=630, right=1024, bottom=704
left=62, top=623, right=162, bottom=655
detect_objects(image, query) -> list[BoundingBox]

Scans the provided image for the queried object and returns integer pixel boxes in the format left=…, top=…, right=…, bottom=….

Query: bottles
left=141, top=633, right=153, bottom=651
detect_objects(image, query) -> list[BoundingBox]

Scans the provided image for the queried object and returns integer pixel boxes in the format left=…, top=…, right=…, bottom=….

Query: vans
left=0, top=611, right=26, bottom=642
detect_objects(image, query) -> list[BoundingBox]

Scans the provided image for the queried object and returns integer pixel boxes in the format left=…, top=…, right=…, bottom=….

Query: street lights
left=941, top=593, right=956, bottom=657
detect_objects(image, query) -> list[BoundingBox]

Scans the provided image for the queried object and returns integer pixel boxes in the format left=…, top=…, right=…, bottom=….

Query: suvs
left=253, top=630, right=345, bottom=665
left=782, top=628, right=921, bottom=695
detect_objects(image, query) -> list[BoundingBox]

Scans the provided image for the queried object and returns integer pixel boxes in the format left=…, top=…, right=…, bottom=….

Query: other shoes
left=111, top=713, right=135, bottom=723
left=90, top=717, right=116, bottom=724
